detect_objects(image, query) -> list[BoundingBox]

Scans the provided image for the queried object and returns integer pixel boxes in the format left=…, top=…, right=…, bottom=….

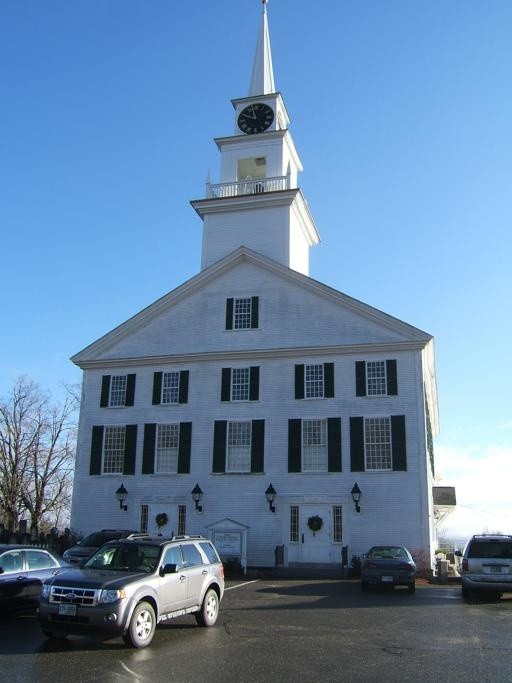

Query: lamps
left=114, top=481, right=129, bottom=511
left=264, top=482, right=278, bottom=512
left=350, top=481, right=362, bottom=512
left=191, top=482, right=204, bottom=512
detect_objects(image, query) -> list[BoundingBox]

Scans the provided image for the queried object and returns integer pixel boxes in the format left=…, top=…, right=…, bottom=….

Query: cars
left=0, top=547, right=78, bottom=621
left=359, top=546, right=416, bottom=593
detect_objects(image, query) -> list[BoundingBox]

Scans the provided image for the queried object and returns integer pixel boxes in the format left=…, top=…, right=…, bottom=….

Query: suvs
left=41, top=529, right=226, bottom=649
left=454, top=535, right=512, bottom=597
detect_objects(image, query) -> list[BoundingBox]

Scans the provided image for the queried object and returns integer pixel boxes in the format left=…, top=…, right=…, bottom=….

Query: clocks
left=237, top=102, right=275, bottom=134
